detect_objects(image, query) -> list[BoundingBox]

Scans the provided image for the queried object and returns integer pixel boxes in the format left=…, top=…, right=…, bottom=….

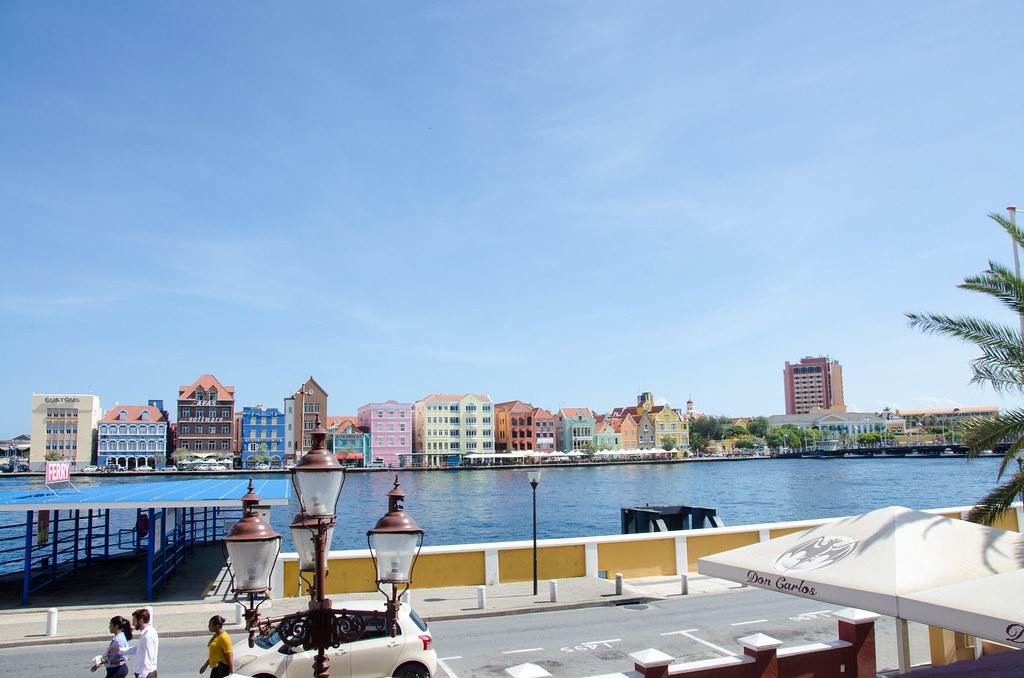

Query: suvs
left=106, top=465, right=118, bottom=471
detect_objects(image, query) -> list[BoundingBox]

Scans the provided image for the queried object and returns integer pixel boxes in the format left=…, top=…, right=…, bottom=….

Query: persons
left=90, top=616, right=133, bottom=678
left=108, top=609, right=159, bottom=678
left=199, top=615, right=235, bottom=678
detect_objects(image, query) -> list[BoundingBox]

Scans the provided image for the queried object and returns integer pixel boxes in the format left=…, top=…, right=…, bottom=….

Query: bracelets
left=108, top=660, right=110, bottom=665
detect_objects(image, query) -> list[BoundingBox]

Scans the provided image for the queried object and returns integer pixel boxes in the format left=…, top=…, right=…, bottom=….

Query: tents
left=697, top=504, right=1024, bottom=678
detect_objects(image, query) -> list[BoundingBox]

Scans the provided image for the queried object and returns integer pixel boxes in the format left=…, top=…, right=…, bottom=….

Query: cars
left=0, top=463, right=9, bottom=470
left=283, top=464, right=297, bottom=469
left=161, top=465, right=177, bottom=471
left=81, top=466, right=98, bottom=472
left=17, top=465, right=28, bottom=472
left=982, top=449, right=993, bottom=454
left=712, top=452, right=721, bottom=457
left=783, top=448, right=790, bottom=452
left=255, top=464, right=269, bottom=470
left=212, top=465, right=226, bottom=470
left=228, top=600, right=437, bottom=678
left=137, top=465, right=152, bottom=471
left=944, top=448, right=953, bottom=454
left=194, top=464, right=208, bottom=470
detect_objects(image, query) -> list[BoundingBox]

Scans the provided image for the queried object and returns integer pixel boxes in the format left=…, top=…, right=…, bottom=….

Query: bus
left=178, top=462, right=233, bottom=471
left=368, top=461, right=383, bottom=467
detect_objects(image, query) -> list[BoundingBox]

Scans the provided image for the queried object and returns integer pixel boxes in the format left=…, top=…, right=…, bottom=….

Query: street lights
left=784, top=435, right=787, bottom=454
left=527, top=468, right=541, bottom=595
left=72, top=456, right=76, bottom=472
left=221, top=412, right=426, bottom=678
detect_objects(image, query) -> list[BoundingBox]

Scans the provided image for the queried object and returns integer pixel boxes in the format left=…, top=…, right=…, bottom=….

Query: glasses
left=209, top=624, right=215, bottom=627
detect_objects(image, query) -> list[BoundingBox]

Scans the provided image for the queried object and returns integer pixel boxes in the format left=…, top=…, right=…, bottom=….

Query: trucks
left=547, top=457, right=570, bottom=462
